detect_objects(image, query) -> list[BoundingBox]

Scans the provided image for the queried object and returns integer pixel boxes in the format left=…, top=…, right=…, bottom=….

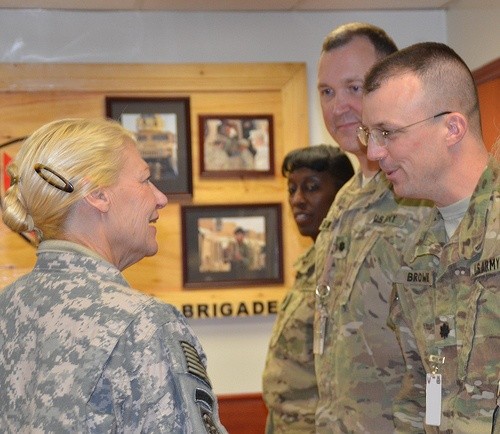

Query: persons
left=0, top=116, right=231, bottom=433
left=224, top=227, right=252, bottom=270
left=313, top=21, right=434, bottom=434
left=249, top=130, right=269, bottom=169
left=362, top=40, right=500, bottom=433
left=261, top=143, right=356, bottom=434
left=216, top=116, right=256, bottom=170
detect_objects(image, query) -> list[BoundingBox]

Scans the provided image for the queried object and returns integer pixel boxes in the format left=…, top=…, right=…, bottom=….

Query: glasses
left=357, top=110, right=453, bottom=147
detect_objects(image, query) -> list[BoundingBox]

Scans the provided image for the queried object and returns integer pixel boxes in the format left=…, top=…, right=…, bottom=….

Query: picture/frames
left=181, top=202, right=286, bottom=291
left=199, top=113, right=276, bottom=180
left=105, top=96, right=192, bottom=200
